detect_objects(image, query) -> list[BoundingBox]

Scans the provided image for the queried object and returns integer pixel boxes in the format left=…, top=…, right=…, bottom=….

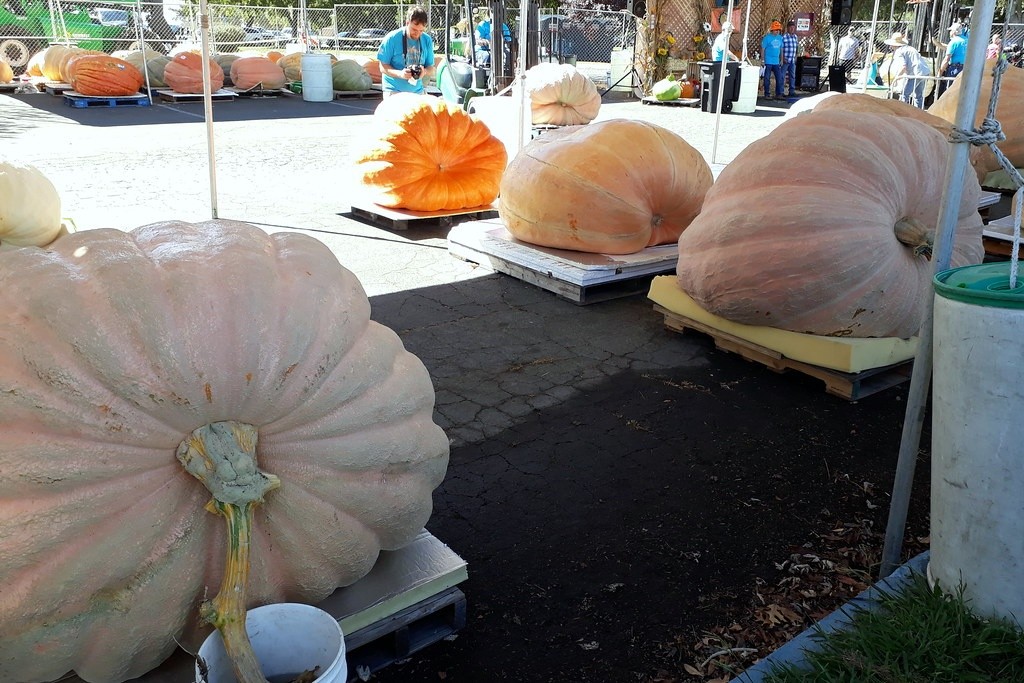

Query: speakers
left=832, top=0, right=853, bottom=25
left=627, top=0, right=647, bottom=21
left=829, top=65, right=846, bottom=93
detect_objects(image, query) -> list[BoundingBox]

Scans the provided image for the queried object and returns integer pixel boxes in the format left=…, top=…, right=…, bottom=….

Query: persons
left=712, top=21, right=740, bottom=62
left=836, top=23, right=1001, bottom=109
left=760, top=21, right=798, bottom=101
left=377, top=6, right=435, bottom=100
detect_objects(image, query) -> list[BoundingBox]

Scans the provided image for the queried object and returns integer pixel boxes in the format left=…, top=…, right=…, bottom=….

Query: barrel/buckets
left=929, top=254, right=1024, bottom=639
left=300, top=52, right=333, bottom=102
left=195, top=601, right=350, bottom=683
left=730, top=64, right=761, bottom=113
left=829, top=65, right=846, bottom=94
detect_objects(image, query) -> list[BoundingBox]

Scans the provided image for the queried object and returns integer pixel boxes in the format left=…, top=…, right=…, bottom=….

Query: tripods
left=819, top=25, right=854, bottom=90
left=601, top=23, right=645, bottom=97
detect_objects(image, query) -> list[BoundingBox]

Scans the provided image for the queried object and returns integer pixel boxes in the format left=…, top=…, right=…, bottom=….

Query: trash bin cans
left=697, top=58, right=742, bottom=114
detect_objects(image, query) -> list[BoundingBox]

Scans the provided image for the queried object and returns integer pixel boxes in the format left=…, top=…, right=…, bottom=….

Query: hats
left=769, top=21, right=782, bottom=31
left=946, top=23, right=963, bottom=30
left=884, top=31, right=908, bottom=46
left=787, top=22, right=796, bottom=27
left=722, top=21, right=736, bottom=30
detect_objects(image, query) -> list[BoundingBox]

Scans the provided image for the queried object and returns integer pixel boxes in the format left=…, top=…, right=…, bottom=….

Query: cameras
left=408, top=65, right=422, bottom=79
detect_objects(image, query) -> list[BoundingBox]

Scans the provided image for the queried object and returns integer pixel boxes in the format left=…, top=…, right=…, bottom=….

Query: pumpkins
left=1, top=50, right=1024, bottom=683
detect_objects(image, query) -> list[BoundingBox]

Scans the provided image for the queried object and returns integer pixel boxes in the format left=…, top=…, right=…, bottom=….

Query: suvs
left=357, top=28, right=392, bottom=48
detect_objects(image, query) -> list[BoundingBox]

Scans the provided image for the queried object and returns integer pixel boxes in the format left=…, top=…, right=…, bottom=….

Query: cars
left=90, top=4, right=320, bottom=54
left=327, top=31, right=358, bottom=50
